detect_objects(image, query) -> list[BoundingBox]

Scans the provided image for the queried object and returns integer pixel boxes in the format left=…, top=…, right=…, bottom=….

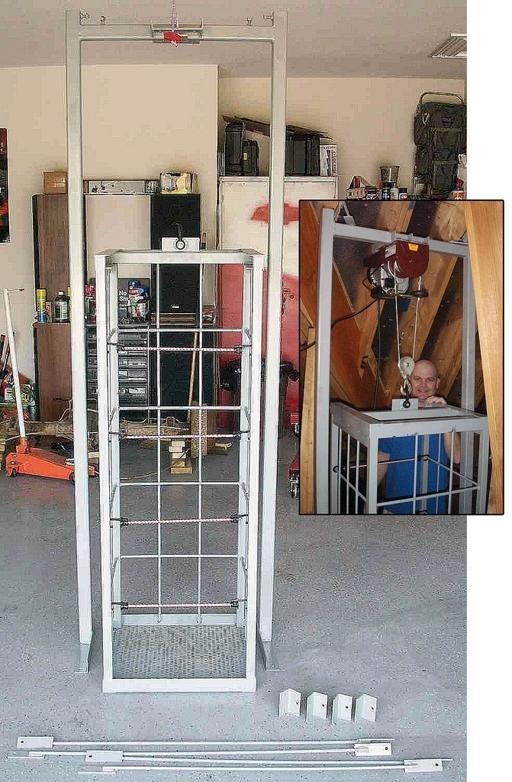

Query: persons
left=374, top=359, right=458, bottom=513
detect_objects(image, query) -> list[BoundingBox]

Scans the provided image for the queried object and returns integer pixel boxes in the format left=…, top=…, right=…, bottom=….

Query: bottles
left=365, top=165, right=408, bottom=200
left=36, top=278, right=150, bottom=325
left=202, top=233, right=207, bottom=249
left=449, top=181, right=464, bottom=200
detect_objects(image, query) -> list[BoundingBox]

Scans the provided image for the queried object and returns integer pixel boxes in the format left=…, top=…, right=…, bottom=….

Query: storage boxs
left=44, top=171, right=68, bottom=194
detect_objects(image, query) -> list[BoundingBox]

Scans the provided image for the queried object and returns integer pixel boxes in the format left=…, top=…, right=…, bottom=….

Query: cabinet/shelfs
left=216, top=175, right=339, bottom=431
left=85, top=322, right=150, bottom=424
